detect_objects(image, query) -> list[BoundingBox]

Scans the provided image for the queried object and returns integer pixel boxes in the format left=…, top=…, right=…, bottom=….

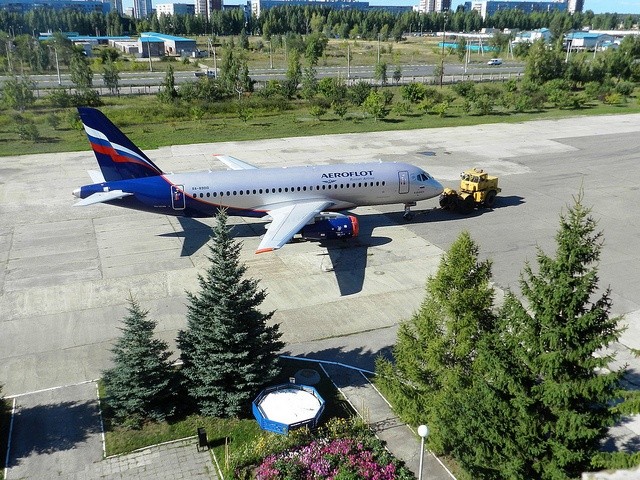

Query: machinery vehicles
left=440, top=168, right=501, bottom=215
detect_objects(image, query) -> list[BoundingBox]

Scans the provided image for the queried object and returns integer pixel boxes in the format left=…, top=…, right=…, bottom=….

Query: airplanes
left=72, top=106, right=444, bottom=254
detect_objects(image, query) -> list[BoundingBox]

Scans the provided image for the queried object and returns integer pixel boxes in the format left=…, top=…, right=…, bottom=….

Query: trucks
left=195, top=70, right=215, bottom=78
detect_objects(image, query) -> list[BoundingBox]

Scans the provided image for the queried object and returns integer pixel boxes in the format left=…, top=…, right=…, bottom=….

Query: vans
left=487, top=59, right=502, bottom=65
